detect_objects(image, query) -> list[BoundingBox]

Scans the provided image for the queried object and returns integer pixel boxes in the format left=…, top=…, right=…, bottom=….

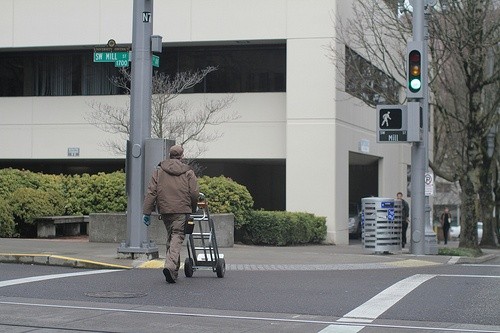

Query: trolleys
left=184, top=193, right=226, bottom=278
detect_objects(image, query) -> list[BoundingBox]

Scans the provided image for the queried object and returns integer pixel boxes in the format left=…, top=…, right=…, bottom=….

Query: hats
left=170, top=145, right=185, bottom=158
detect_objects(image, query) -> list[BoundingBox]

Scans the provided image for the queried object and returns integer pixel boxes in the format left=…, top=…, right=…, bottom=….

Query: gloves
left=142, top=214, right=151, bottom=226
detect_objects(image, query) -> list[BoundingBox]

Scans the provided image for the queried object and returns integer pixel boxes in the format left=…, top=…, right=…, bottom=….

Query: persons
left=396, top=192, right=409, bottom=247
left=440, top=207, right=452, bottom=245
left=143, top=146, right=199, bottom=283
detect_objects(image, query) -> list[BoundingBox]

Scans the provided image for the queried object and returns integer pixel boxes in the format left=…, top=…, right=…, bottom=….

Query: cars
left=448, top=218, right=482, bottom=243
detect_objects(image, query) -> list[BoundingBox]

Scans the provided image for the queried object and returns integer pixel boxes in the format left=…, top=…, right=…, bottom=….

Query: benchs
left=32, top=216, right=88, bottom=238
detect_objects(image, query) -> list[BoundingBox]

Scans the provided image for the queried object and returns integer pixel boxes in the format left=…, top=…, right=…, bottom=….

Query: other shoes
left=162, top=268, right=177, bottom=283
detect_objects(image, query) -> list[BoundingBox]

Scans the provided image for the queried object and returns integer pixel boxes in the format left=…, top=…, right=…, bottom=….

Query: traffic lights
left=376, top=104, right=405, bottom=131
left=406, top=46, right=424, bottom=99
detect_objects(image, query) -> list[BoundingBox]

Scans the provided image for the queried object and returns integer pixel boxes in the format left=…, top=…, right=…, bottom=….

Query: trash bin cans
left=437, top=226, right=450, bottom=241
left=361, top=197, right=402, bottom=253
